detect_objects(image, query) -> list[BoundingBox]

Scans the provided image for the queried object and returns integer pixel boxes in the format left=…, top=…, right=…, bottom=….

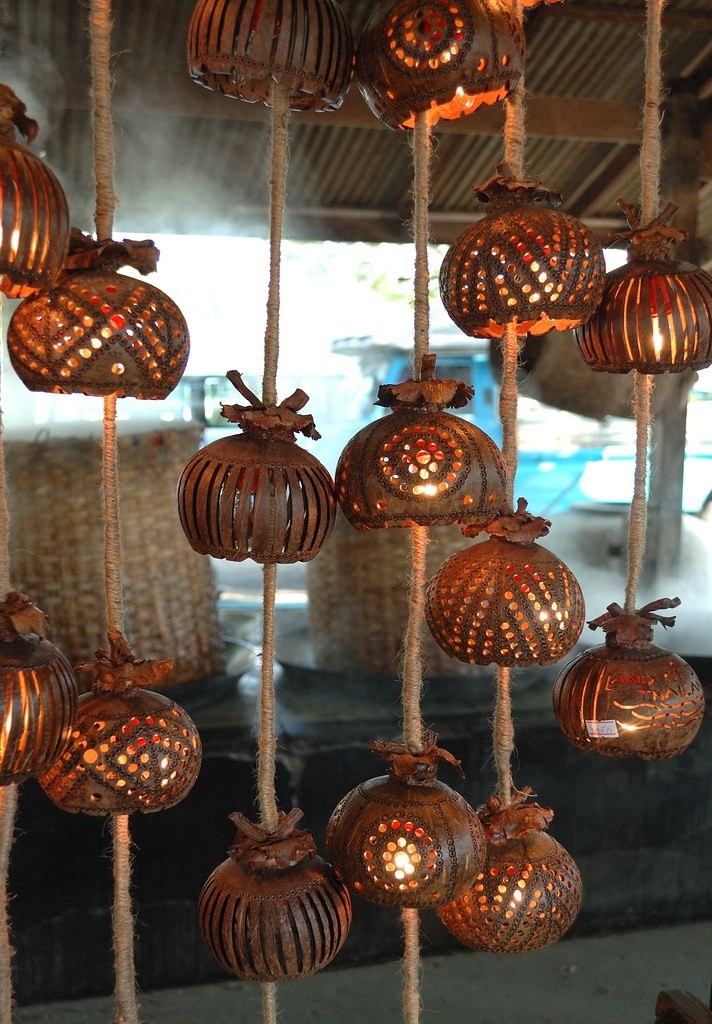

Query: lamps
left=0, top=2, right=712, bottom=1024
